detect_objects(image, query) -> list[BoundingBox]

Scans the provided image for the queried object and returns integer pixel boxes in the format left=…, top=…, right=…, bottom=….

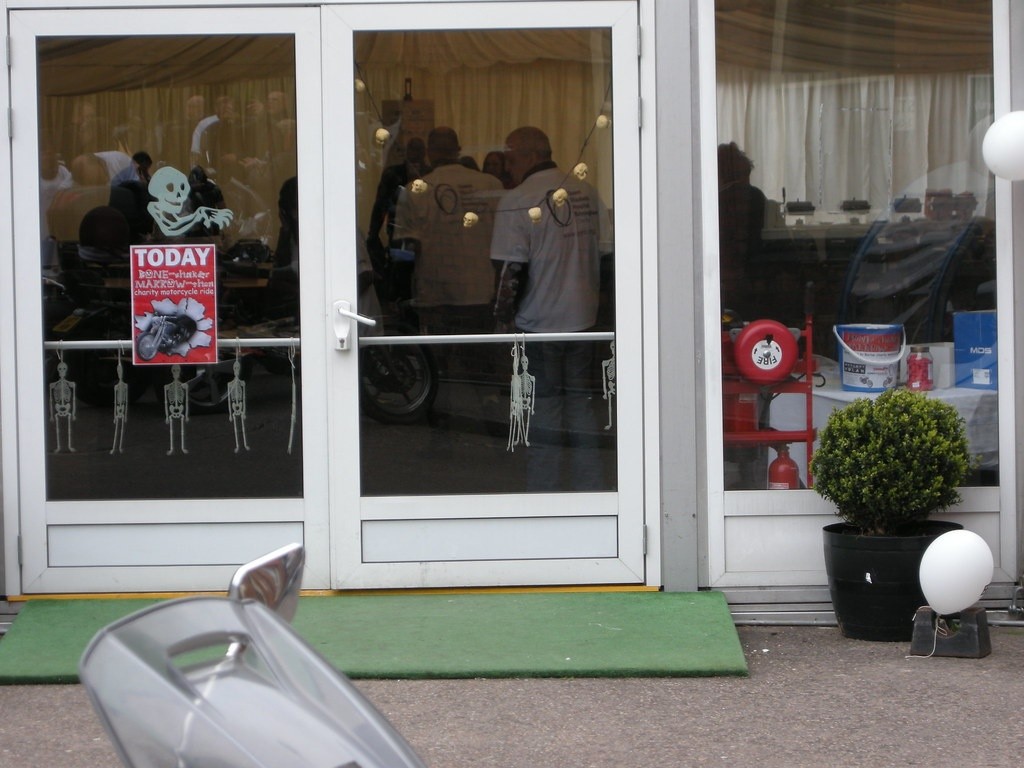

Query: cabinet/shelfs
left=766, top=372, right=998, bottom=489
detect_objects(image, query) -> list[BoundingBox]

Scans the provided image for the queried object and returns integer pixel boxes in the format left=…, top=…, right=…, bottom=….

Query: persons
left=182, top=164, right=228, bottom=248
left=265, top=173, right=302, bottom=328
left=601, top=338, right=618, bottom=432
left=37, top=89, right=296, bottom=245
left=715, top=139, right=771, bottom=329
left=364, top=125, right=617, bottom=495
left=108, top=146, right=161, bottom=244
left=49, top=362, right=249, bottom=454
left=506, top=352, right=538, bottom=446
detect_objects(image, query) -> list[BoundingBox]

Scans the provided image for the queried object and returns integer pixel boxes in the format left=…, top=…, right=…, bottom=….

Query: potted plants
left=808, top=389, right=965, bottom=642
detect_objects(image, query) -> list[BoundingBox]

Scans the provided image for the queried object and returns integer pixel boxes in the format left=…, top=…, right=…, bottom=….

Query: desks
left=762, top=209, right=926, bottom=240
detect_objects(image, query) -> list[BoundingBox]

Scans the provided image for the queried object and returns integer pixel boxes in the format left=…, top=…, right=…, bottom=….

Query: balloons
left=918, top=529, right=995, bottom=615
left=980, top=110, right=1024, bottom=182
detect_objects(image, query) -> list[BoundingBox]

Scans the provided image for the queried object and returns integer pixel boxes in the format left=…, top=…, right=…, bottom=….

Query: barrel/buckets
left=833, top=322, right=906, bottom=393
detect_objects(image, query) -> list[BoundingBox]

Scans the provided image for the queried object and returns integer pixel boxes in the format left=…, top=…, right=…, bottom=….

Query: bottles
left=907, top=345, right=934, bottom=392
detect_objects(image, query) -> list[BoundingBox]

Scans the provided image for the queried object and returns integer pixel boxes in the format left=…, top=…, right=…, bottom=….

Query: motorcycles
left=43, top=208, right=442, bottom=426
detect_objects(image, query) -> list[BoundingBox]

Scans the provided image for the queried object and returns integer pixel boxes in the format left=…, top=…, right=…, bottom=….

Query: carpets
left=0, top=591, right=750, bottom=685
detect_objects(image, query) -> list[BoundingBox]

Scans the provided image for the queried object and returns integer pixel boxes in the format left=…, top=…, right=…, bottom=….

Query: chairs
left=842, top=200, right=872, bottom=210
left=894, top=198, right=924, bottom=212
left=785, top=198, right=815, bottom=212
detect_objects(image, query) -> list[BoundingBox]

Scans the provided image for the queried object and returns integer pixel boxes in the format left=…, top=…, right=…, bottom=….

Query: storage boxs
left=899, top=342, right=954, bottom=389
left=953, top=309, right=998, bottom=390
